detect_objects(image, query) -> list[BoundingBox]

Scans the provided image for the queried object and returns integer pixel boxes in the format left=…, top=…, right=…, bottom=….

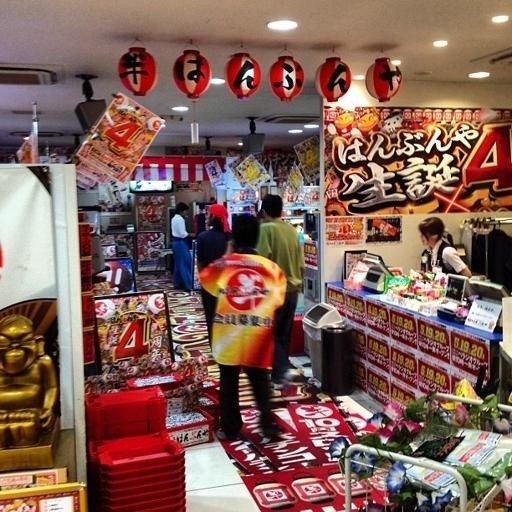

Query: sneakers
left=217, top=430, right=239, bottom=441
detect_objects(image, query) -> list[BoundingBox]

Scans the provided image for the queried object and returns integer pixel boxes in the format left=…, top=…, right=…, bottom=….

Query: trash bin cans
left=302, top=302, right=350, bottom=382
left=321, top=324, right=355, bottom=396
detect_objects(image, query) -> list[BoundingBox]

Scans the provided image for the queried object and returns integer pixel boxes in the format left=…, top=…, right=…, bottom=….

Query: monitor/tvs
left=464, top=276, right=511, bottom=301
left=363, top=255, right=397, bottom=279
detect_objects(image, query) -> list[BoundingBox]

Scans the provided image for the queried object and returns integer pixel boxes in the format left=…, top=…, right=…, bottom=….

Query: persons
left=172, top=193, right=230, bottom=351
left=254, top=195, right=305, bottom=378
left=418, top=217, right=472, bottom=278
left=199, top=212, right=287, bottom=439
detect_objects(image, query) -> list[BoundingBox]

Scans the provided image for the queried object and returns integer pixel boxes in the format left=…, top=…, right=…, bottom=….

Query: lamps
left=191, top=101, right=199, bottom=145
left=75, top=74, right=106, bottom=128
left=245, top=117, right=265, bottom=153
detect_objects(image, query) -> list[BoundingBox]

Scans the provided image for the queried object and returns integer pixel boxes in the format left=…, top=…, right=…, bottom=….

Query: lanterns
left=365, top=57, right=403, bottom=103
left=226, top=51, right=261, bottom=102
left=269, top=56, right=304, bottom=101
left=118, top=45, right=156, bottom=97
left=315, top=56, right=351, bottom=102
left=172, top=48, right=210, bottom=100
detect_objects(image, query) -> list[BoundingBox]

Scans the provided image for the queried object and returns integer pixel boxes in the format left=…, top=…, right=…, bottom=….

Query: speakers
left=243, top=132, right=265, bottom=155
left=74, top=99, right=107, bottom=133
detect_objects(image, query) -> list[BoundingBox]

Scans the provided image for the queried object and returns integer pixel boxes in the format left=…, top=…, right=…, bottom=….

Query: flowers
left=330, top=379, right=512, bottom=512
